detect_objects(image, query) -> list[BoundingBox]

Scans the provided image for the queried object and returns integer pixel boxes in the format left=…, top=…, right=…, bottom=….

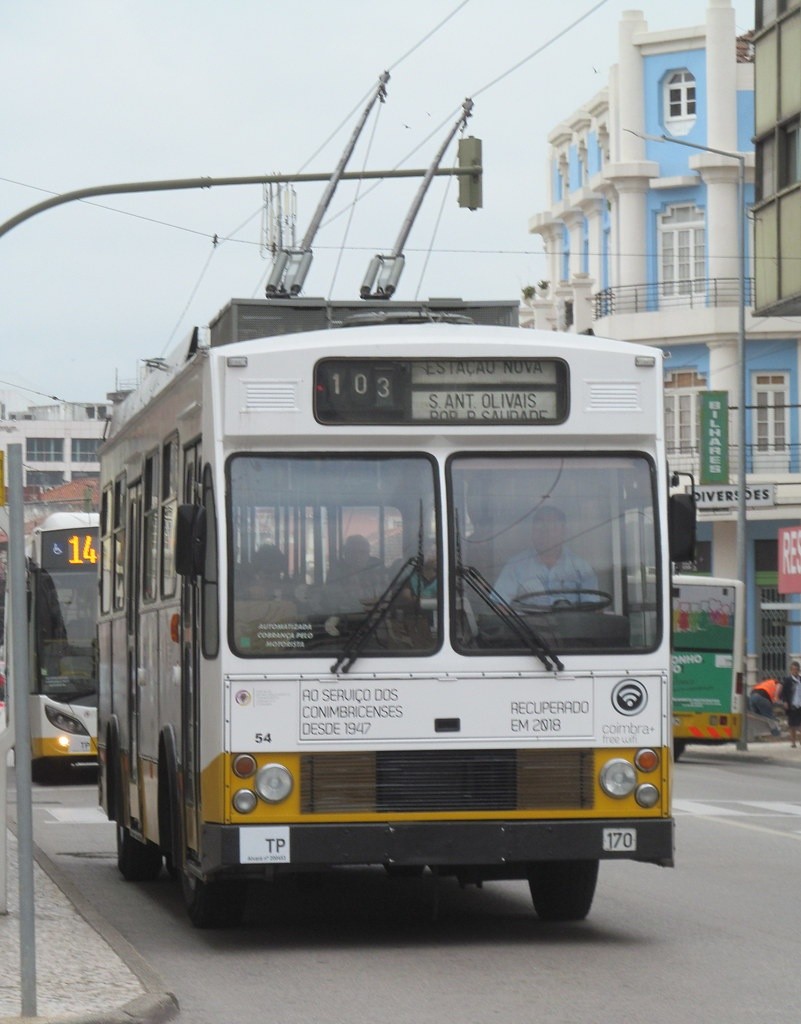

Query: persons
left=392, top=539, right=437, bottom=604
left=781, top=661, right=801, bottom=747
left=750, top=679, right=783, bottom=736
left=489, top=505, right=602, bottom=612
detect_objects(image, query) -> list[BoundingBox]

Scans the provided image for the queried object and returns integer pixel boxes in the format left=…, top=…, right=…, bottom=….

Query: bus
left=1, top=510, right=121, bottom=783
left=94, top=67, right=702, bottom=935
left=617, top=566, right=747, bottom=763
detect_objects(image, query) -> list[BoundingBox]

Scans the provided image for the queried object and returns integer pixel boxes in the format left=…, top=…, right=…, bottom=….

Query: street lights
left=619, top=115, right=753, bottom=752
